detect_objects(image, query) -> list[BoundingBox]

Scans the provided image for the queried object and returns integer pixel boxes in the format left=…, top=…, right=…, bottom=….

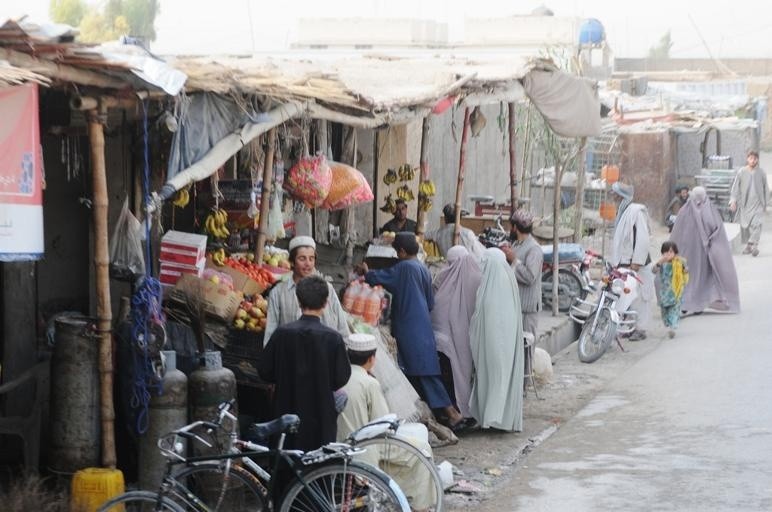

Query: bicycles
left=95, top=413, right=411, bottom=512
left=162, top=399, right=443, bottom=512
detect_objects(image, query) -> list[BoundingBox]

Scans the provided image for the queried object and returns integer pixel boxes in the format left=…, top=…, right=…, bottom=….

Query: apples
left=207, top=254, right=278, bottom=289
left=383, top=230, right=395, bottom=239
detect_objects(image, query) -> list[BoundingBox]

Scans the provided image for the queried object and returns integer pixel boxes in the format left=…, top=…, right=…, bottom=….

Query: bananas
left=202, top=205, right=230, bottom=238
left=213, top=247, right=225, bottom=268
left=173, top=186, right=189, bottom=209
left=423, top=239, right=441, bottom=258
left=378, top=165, right=436, bottom=212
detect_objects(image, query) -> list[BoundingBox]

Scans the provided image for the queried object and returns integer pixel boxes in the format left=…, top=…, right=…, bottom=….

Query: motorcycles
left=476, top=212, right=599, bottom=313
left=566, top=258, right=643, bottom=364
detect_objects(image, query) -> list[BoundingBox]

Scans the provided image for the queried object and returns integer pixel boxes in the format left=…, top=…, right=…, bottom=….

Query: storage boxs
left=149, top=226, right=265, bottom=328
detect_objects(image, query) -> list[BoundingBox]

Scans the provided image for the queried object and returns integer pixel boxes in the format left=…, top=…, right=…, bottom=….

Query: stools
left=519, top=329, right=547, bottom=404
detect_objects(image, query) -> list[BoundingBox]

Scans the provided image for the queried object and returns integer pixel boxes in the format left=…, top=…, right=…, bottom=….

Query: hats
left=512, top=210, right=533, bottom=226
left=348, top=333, right=376, bottom=352
left=395, top=232, right=416, bottom=244
left=289, top=236, right=316, bottom=250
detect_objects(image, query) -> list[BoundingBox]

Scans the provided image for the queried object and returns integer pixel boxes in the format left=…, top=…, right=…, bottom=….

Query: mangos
left=234, top=293, right=269, bottom=332
left=245, top=253, right=291, bottom=270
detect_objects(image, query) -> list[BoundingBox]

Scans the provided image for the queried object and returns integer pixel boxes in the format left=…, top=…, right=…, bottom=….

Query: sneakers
left=743, top=246, right=759, bottom=255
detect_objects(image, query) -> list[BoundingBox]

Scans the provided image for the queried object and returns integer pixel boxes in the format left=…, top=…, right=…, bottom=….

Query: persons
left=501, top=212, right=544, bottom=398
left=433, top=203, right=486, bottom=262
left=652, top=241, right=688, bottom=339
left=335, top=333, right=436, bottom=512
left=729, top=150, right=772, bottom=257
left=262, top=235, right=352, bottom=353
left=260, top=275, right=352, bottom=512
left=665, top=186, right=689, bottom=230
left=612, top=181, right=653, bottom=340
left=378, top=198, right=418, bottom=237
left=670, top=187, right=740, bottom=316
left=352, top=231, right=523, bottom=434
